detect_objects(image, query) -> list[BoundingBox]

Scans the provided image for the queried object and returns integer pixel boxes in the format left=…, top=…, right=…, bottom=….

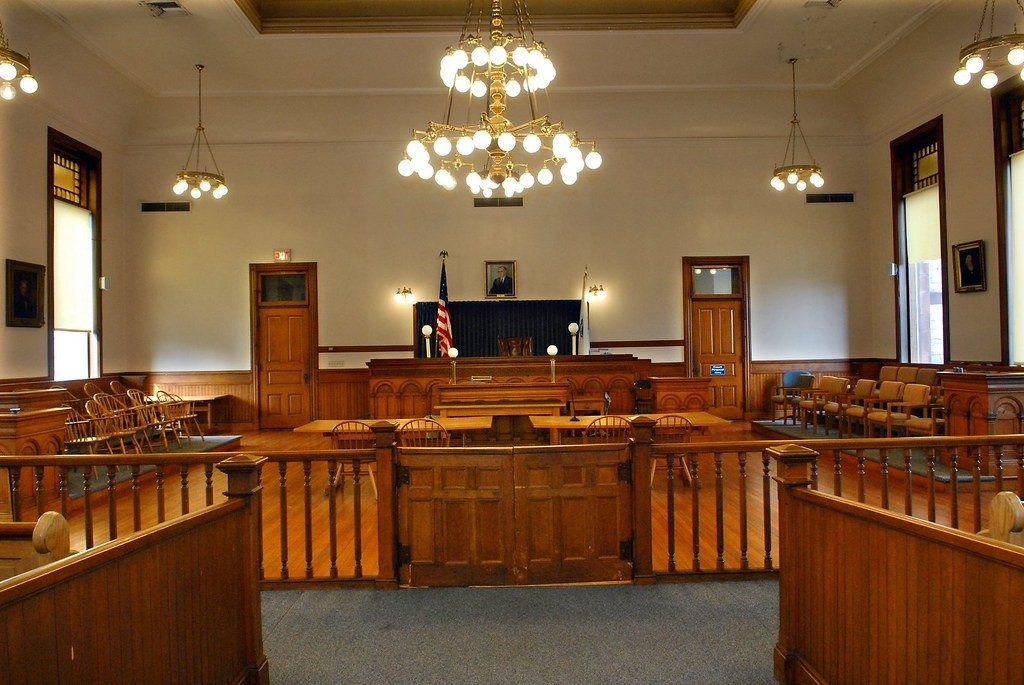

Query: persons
left=489, top=266, right=512, bottom=294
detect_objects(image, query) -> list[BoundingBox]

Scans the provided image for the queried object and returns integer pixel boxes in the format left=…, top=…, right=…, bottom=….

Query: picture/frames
left=5, top=258, right=46, bottom=328
left=952, top=240, right=987, bottom=294
left=484, top=260, right=517, bottom=299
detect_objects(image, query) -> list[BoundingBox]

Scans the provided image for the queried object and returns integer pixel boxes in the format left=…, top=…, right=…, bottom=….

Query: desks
left=144, top=394, right=229, bottom=429
left=293, top=416, right=491, bottom=495
left=530, top=412, right=731, bottom=488
left=434, top=404, right=565, bottom=447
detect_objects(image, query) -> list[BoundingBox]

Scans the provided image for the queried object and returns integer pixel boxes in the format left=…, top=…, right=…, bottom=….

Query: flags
left=578, top=276, right=590, bottom=355
left=436, top=258, right=452, bottom=358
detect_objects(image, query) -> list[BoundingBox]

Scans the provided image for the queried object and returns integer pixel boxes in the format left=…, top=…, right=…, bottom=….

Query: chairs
left=897, top=367, right=920, bottom=412
left=824, top=379, right=877, bottom=439
left=400, top=418, right=450, bottom=448
left=650, top=415, right=694, bottom=486
left=792, top=376, right=837, bottom=429
left=772, top=374, right=814, bottom=425
left=904, top=403, right=945, bottom=463
left=799, top=378, right=850, bottom=435
left=332, top=421, right=378, bottom=500
left=855, top=366, right=899, bottom=409
left=498, top=336, right=533, bottom=356
left=50, top=380, right=204, bottom=479
left=776, top=369, right=813, bottom=424
left=916, top=369, right=940, bottom=418
left=582, top=415, right=631, bottom=443
left=847, top=381, right=905, bottom=439
left=868, top=383, right=930, bottom=438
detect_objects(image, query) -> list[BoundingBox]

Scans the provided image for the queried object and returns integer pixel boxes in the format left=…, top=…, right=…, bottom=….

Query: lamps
left=0, top=21, right=39, bottom=99
left=422, top=326, right=432, bottom=357
left=397, top=0, right=603, bottom=198
left=953, top=0, right=1024, bottom=88
left=547, top=345, right=558, bottom=383
left=770, top=59, right=825, bottom=192
left=395, top=287, right=414, bottom=303
left=448, top=347, right=458, bottom=384
left=174, top=65, right=228, bottom=199
left=587, top=285, right=605, bottom=301
left=568, top=323, right=579, bottom=355
